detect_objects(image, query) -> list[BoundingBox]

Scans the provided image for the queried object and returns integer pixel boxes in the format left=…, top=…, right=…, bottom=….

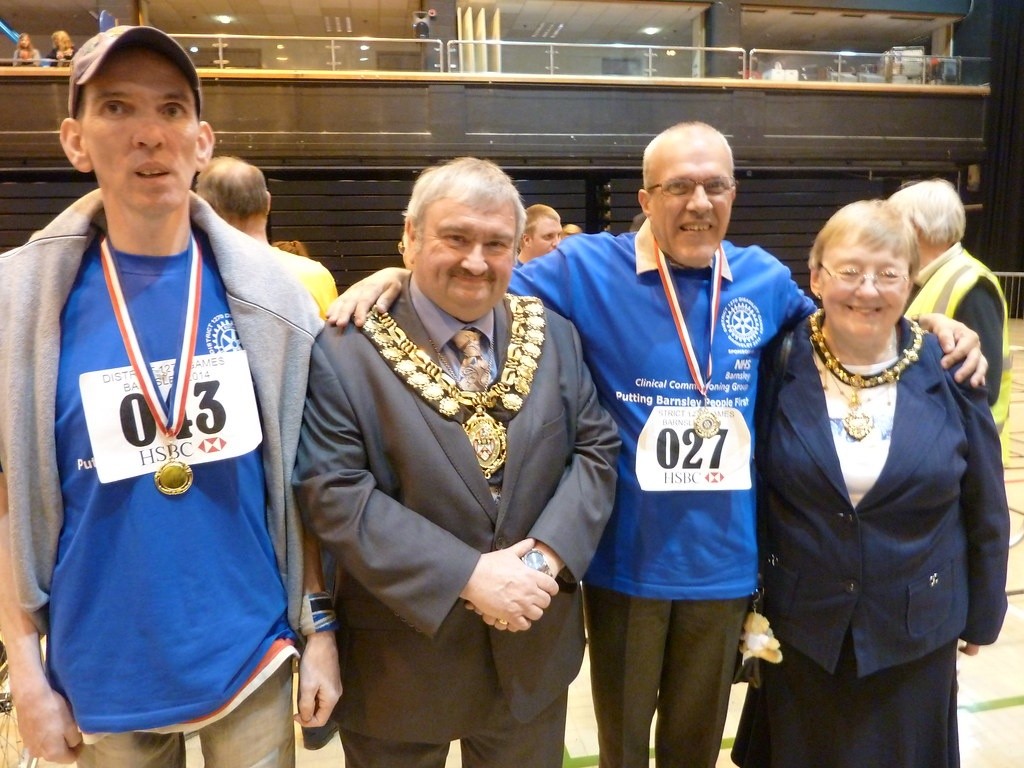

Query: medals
left=693, top=409, right=721, bottom=439
left=464, top=412, right=507, bottom=480
left=841, top=409, right=874, bottom=438
left=155, top=458, right=193, bottom=494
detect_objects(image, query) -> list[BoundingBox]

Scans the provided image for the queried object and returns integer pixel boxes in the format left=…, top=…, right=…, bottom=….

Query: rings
left=496, top=618, right=509, bottom=625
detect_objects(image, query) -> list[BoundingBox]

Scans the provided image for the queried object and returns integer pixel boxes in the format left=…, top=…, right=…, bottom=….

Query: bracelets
left=299, top=592, right=340, bottom=635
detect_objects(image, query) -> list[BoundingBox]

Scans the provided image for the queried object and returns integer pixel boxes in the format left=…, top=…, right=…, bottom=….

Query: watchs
left=520, top=549, right=554, bottom=576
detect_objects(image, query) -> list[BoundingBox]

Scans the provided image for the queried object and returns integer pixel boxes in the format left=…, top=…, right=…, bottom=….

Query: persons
left=730, top=198, right=1009, bottom=768
left=328, top=122, right=988, bottom=768
left=0, top=26, right=344, bottom=768
left=193, top=157, right=580, bottom=325
left=49, top=31, right=78, bottom=68
left=888, top=179, right=1008, bottom=472
left=293, top=158, right=623, bottom=768
left=13, top=32, right=40, bottom=67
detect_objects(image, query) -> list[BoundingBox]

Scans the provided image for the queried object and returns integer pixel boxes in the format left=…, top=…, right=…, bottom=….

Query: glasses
left=822, top=264, right=909, bottom=288
left=645, top=176, right=734, bottom=196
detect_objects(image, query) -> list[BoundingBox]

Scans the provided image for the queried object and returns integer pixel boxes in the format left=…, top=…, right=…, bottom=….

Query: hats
left=68, top=25, right=202, bottom=121
